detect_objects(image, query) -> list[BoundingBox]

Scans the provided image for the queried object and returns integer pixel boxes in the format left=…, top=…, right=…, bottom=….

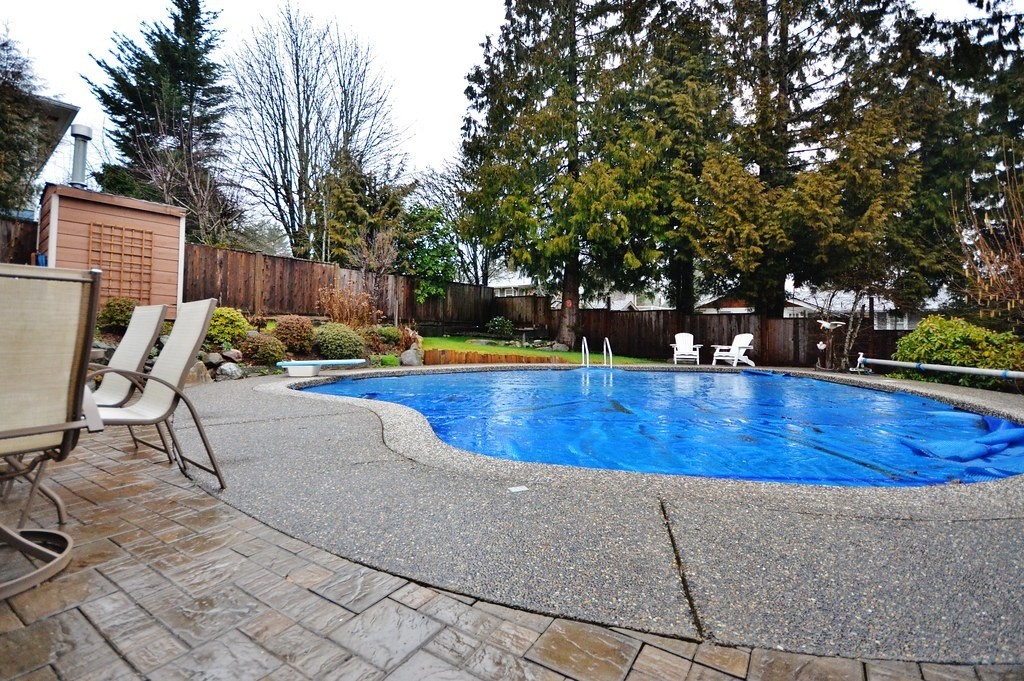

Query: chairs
left=711, top=333, right=755, bottom=367
left=88, top=304, right=173, bottom=462
left=0, top=259, right=102, bottom=530
left=670, top=332, right=703, bottom=365
left=86, top=298, right=227, bottom=488
